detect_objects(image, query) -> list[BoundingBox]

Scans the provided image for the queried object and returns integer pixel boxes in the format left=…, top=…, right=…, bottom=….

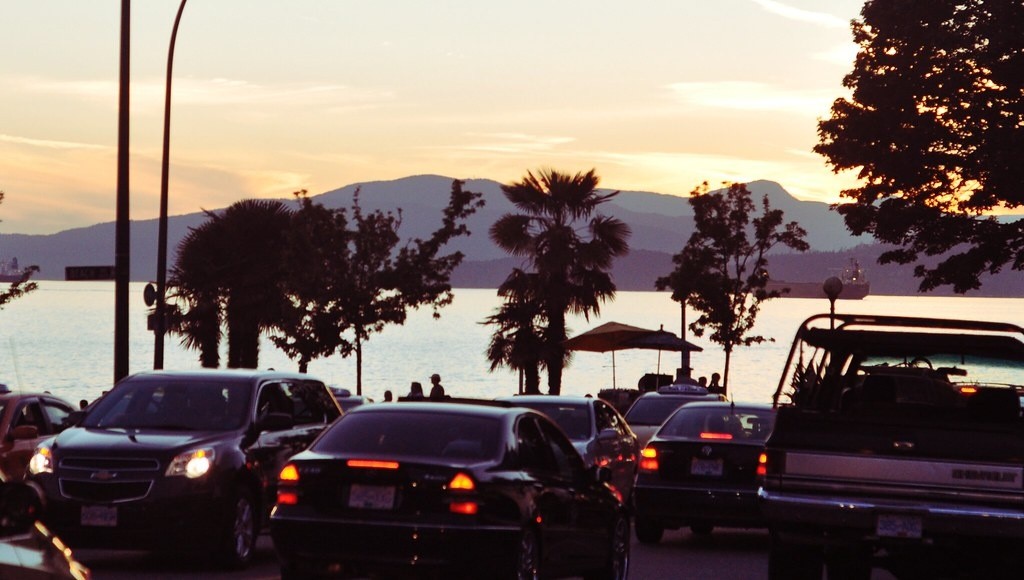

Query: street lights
left=824, top=277, right=843, bottom=367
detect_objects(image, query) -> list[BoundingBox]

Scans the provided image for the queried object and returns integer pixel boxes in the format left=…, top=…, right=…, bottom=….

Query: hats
left=429, top=374, right=440, bottom=378
left=0, top=384, right=11, bottom=393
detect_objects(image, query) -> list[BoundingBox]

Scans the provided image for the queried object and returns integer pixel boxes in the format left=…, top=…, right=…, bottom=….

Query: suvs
left=26, top=369, right=345, bottom=566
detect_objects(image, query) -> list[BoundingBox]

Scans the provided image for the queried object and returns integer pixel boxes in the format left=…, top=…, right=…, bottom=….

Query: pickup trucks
left=757, top=315, right=1024, bottom=580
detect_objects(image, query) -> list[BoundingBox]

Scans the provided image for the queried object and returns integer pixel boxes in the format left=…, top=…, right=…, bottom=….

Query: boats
left=752, top=257, right=869, bottom=301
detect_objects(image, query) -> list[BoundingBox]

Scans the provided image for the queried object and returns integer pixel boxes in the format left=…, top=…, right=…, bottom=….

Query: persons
left=707, top=373, right=724, bottom=393
left=383, top=391, right=392, bottom=402
left=430, top=374, right=444, bottom=398
left=699, top=377, right=707, bottom=388
left=80, top=400, right=88, bottom=410
left=408, top=382, right=423, bottom=398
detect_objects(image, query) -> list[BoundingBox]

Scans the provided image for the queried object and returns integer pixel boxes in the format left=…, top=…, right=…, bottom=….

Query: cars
left=0, top=383, right=82, bottom=540
left=495, top=394, right=640, bottom=499
left=634, top=397, right=783, bottom=542
left=623, top=383, right=725, bottom=456
left=268, top=401, right=629, bottom=580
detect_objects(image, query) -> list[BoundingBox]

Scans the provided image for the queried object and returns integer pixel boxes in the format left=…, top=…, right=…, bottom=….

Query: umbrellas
left=560, top=323, right=702, bottom=391
